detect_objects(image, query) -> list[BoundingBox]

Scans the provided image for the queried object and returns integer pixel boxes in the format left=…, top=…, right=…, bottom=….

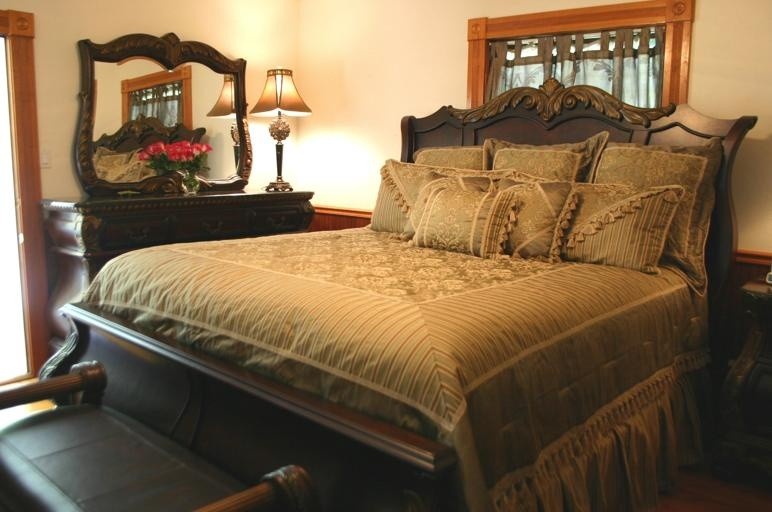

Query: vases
left=181, top=168, right=200, bottom=194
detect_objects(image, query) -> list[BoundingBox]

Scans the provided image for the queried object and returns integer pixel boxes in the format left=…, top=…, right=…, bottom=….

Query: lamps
left=250, top=64, right=313, bottom=193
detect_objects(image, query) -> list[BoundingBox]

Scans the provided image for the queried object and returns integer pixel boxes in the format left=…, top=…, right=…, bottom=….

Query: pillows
left=412, top=184, right=518, bottom=259
left=409, top=141, right=489, bottom=174
left=485, top=130, right=611, bottom=181
left=592, top=137, right=722, bottom=295
left=491, top=180, right=577, bottom=264
left=401, top=173, right=502, bottom=257
left=511, top=168, right=684, bottom=275
left=371, top=158, right=511, bottom=241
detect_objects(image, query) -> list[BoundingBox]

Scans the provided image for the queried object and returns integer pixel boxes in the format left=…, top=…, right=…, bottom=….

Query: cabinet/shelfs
left=39, top=190, right=314, bottom=353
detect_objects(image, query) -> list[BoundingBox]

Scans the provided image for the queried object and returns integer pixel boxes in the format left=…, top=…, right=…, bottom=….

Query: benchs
left=0, top=359, right=323, bottom=512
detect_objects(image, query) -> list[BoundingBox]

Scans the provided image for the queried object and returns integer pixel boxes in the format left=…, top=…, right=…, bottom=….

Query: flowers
left=166, top=140, right=212, bottom=191
left=137, top=141, right=179, bottom=175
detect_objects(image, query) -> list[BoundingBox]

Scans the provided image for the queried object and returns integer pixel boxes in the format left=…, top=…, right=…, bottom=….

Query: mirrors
left=74, top=31, right=253, bottom=195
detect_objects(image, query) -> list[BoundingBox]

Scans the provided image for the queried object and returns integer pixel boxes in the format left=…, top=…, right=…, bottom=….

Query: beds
left=36, top=78, right=759, bottom=512
left=91, top=113, right=206, bottom=183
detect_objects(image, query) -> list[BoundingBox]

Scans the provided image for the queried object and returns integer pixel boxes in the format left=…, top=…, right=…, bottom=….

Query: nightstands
left=713, top=272, right=771, bottom=482
